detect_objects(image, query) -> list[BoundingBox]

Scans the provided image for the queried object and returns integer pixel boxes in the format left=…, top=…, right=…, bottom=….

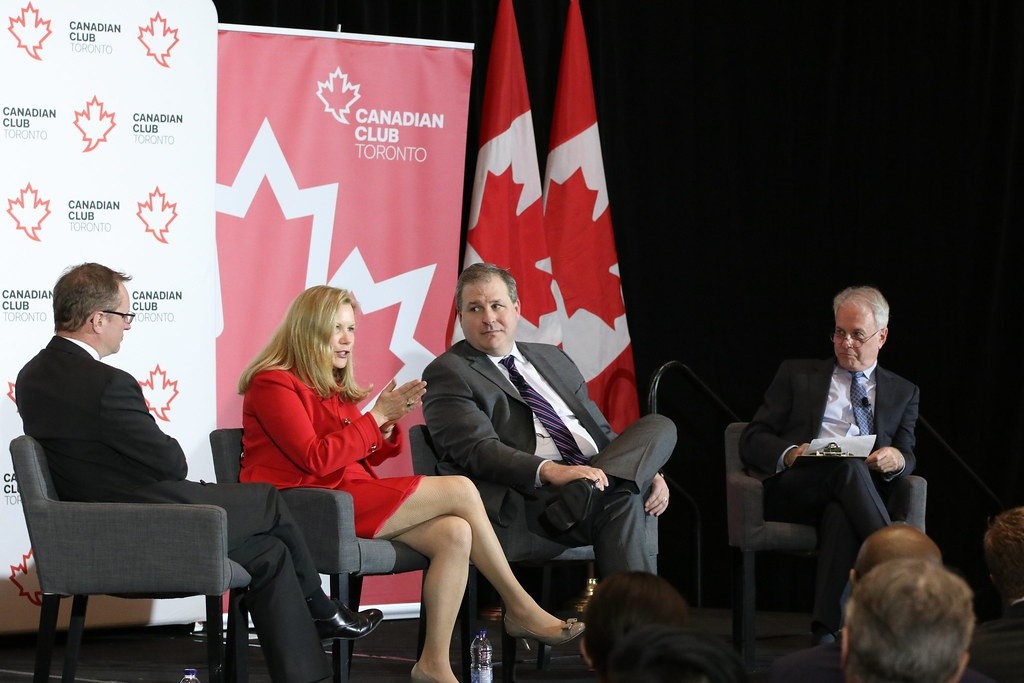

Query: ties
left=848, top=371, right=875, bottom=436
left=498, top=355, right=589, bottom=466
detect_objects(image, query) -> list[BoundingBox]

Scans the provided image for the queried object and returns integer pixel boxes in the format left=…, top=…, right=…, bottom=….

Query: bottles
left=179, top=668, right=202, bottom=683
left=469, top=631, right=492, bottom=683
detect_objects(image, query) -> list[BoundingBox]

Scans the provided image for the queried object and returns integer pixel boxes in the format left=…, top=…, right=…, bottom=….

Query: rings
left=663, top=497, right=668, bottom=502
left=406, top=399, right=411, bottom=406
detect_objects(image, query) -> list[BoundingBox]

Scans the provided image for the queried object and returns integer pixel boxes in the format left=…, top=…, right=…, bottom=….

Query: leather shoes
left=546, top=477, right=601, bottom=531
left=314, top=601, right=383, bottom=646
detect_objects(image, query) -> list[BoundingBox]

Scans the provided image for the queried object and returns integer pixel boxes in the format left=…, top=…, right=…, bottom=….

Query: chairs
left=209, top=427, right=477, bottom=683
left=724, top=422, right=928, bottom=671
left=10, top=435, right=252, bottom=683
left=410, top=423, right=594, bottom=683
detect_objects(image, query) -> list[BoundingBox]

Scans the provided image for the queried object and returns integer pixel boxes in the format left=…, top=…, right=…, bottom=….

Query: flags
left=446, top=0, right=641, bottom=440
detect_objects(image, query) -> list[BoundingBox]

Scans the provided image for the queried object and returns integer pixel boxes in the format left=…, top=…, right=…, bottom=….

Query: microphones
left=862, top=397, right=871, bottom=407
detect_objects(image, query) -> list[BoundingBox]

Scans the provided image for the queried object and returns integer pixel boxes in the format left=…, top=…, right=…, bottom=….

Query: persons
left=421, top=263, right=677, bottom=583
left=577, top=572, right=751, bottom=683
left=15, top=263, right=383, bottom=683
left=237, top=285, right=587, bottom=683
left=769, top=504, right=1024, bottom=683
left=738, top=287, right=920, bottom=646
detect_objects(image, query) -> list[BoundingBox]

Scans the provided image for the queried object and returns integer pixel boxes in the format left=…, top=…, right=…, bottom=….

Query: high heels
left=503, top=614, right=584, bottom=651
left=411, top=663, right=434, bottom=683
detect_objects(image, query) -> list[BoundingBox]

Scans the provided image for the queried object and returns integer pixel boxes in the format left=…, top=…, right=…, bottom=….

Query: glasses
left=830, top=328, right=884, bottom=349
left=91, top=310, right=136, bottom=323
